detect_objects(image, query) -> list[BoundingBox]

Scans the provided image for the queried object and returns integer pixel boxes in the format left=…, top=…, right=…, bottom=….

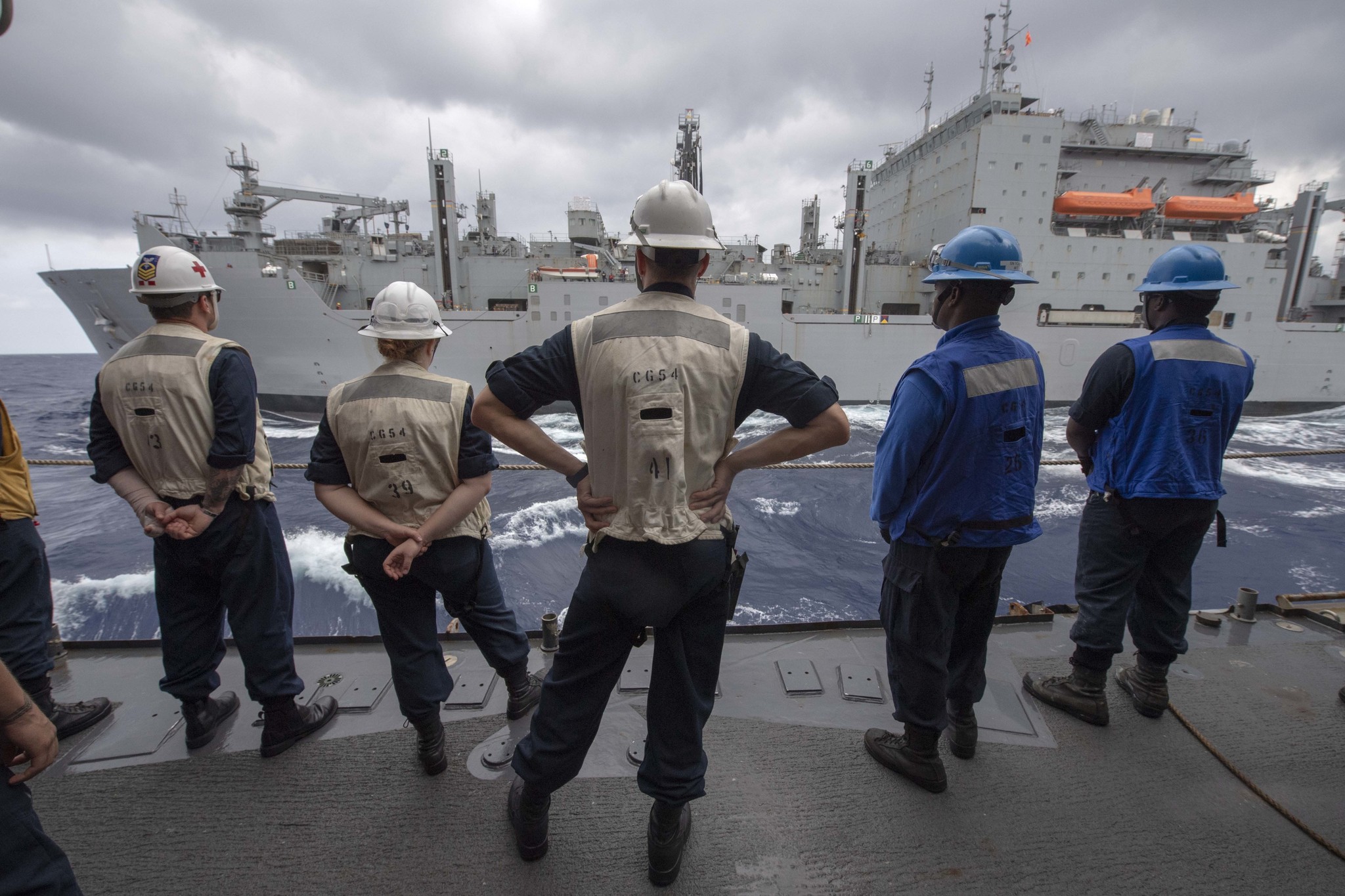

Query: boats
left=1163, top=191, right=1259, bottom=220
left=536, top=265, right=598, bottom=279
left=1054, top=187, right=1154, bottom=217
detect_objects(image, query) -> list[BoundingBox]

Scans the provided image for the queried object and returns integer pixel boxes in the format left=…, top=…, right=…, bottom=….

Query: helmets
left=616, top=179, right=728, bottom=252
left=357, top=281, right=453, bottom=340
left=921, top=226, right=1040, bottom=284
left=337, top=303, right=340, bottom=306
left=129, top=246, right=226, bottom=294
left=1133, top=244, right=1242, bottom=292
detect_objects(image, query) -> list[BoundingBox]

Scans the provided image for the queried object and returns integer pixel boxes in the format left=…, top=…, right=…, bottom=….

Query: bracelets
left=199, top=500, right=221, bottom=519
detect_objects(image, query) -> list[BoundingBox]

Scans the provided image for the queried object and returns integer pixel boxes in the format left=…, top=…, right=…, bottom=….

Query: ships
left=34, top=1, right=1345, bottom=409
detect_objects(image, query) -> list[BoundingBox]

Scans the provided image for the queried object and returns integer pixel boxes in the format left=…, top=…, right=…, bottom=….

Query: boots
left=1022, top=656, right=1110, bottom=727
left=647, top=800, right=691, bottom=886
left=944, top=701, right=978, bottom=760
left=864, top=723, right=948, bottom=793
left=506, top=667, right=550, bottom=720
left=508, top=774, right=551, bottom=861
left=259, top=695, right=338, bottom=758
left=180, top=691, right=240, bottom=748
left=403, top=714, right=448, bottom=775
left=1115, top=650, right=1169, bottom=718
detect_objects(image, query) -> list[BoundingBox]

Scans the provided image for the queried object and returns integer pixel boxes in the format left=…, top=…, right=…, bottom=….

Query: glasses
left=369, top=300, right=437, bottom=336
left=215, top=289, right=222, bottom=302
left=1139, top=292, right=1165, bottom=303
left=928, top=243, right=947, bottom=272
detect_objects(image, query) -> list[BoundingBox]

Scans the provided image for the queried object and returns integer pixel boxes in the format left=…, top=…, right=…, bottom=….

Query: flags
left=1025, top=30, right=1032, bottom=46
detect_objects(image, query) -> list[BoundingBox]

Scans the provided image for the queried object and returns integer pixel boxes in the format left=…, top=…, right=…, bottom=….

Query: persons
left=1022, top=246, right=1257, bottom=728
left=227, top=262, right=233, bottom=268
left=0, top=396, right=111, bottom=741
left=601, top=270, right=607, bottom=282
left=86, top=247, right=341, bottom=752
left=609, top=273, right=614, bottom=282
left=0, top=659, right=82, bottom=896
left=864, top=224, right=1044, bottom=793
left=303, top=281, right=542, bottom=775
left=336, top=303, right=341, bottom=310
left=194, top=236, right=200, bottom=252
left=1299, top=307, right=1308, bottom=324
left=473, top=180, right=851, bottom=888
left=621, top=266, right=627, bottom=282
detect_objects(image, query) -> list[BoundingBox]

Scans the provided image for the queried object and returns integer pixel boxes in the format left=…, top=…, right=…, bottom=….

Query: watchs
left=566, top=462, right=589, bottom=488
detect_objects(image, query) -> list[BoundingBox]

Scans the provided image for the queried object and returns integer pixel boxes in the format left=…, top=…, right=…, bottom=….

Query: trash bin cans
left=716, top=280, right=719, bottom=283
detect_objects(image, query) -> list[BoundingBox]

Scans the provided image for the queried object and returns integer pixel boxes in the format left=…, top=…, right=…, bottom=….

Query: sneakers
left=47, top=696, right=111, bottom=742
